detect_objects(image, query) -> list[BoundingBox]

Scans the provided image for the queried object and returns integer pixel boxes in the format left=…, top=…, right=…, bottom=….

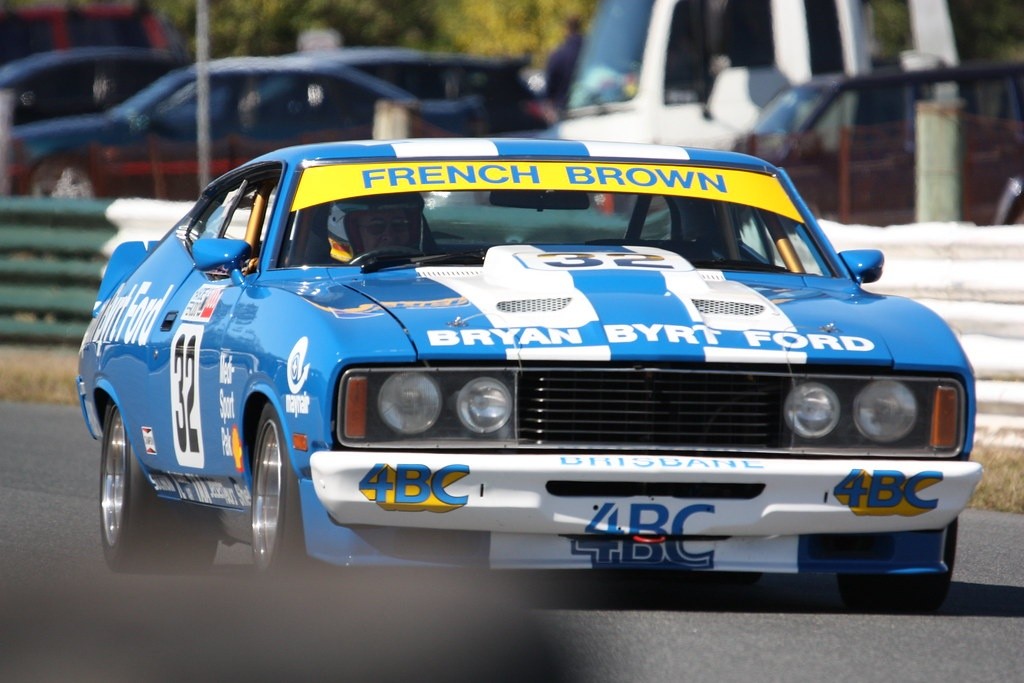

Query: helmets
left=326, top=192, right=425, bottom=262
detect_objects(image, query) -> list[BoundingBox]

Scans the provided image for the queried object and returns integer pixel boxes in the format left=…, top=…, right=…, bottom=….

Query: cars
left=0, top=43, right=562, bottom=202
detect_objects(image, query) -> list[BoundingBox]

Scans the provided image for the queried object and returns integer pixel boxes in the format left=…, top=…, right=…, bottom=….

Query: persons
left=326, top=190, right=425, bottom=268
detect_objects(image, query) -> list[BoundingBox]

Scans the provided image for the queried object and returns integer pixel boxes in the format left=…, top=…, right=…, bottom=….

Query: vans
left=558, top=0, right=962, bottom=152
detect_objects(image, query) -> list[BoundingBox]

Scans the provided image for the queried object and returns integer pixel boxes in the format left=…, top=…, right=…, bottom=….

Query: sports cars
left=80, top=135, right=983, bottom=629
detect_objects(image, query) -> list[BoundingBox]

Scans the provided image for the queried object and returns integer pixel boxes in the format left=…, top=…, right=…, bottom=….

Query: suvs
left=727, top=61, right=1024, bottom=232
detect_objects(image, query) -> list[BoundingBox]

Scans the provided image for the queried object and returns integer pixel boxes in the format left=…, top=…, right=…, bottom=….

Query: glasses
left=345, top=216, right=414, bottom=235
